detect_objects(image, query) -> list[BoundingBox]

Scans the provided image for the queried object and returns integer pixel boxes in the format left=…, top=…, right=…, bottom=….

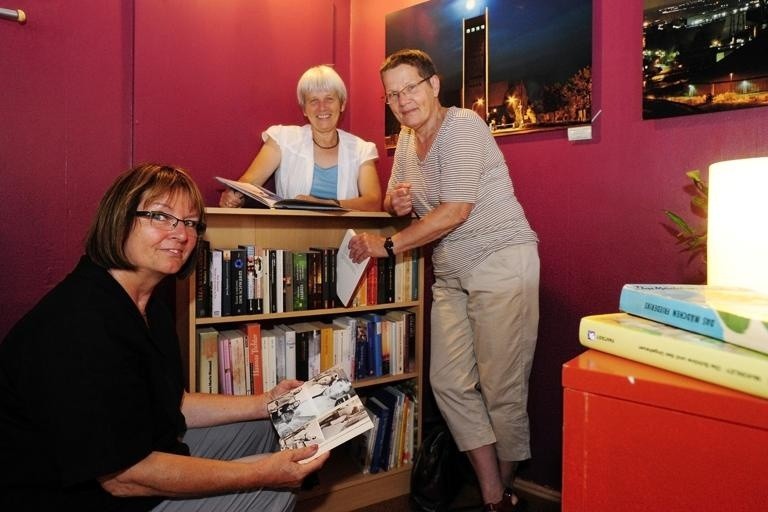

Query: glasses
left=380, top=76, right=430, bottom=104
left=132, top=210, right=206, bottom=236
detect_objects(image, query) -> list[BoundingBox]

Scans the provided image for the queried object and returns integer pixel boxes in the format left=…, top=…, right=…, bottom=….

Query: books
left=197, top=309, right=417, bottom=402
left=266, top=366, right=374, bottom=465
left=620, top=266, right=768, bottom=355
left=578, top=311, right=767, bottom=400
left=197, top=228, right=418, bottom=319
left=214, top=176, right=352, bottom=213
left=333, top=377, right=420, bottom=476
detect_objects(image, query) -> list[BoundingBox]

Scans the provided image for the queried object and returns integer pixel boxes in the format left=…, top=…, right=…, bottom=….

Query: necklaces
left=314, top=131, right=339, bottom=149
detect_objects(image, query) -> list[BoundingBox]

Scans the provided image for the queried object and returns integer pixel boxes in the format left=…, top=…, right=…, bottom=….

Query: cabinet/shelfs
left=178, top=207, right=423, bottom=511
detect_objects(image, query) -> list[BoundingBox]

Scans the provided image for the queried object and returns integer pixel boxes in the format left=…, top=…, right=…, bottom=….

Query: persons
left=220, top=63, right=382, bottom=212
left=348, top=49, right=542, bottom=511
left=269, top=368, right=358, bottom=462
left=2, top=161, right=331, bottom=512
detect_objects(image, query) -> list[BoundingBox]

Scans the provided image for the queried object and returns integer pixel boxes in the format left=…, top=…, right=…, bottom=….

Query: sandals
left=483, top=489, right=521, bottom=511
